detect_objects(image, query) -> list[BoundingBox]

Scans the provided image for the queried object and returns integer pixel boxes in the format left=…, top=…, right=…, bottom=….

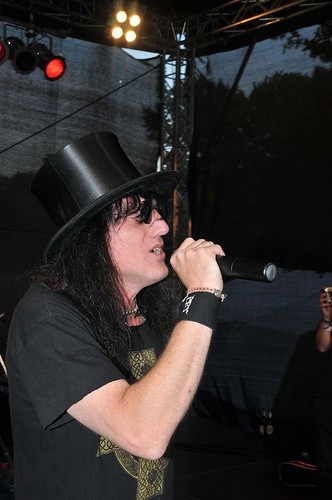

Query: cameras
left=321, top=287, right=332, bottom=305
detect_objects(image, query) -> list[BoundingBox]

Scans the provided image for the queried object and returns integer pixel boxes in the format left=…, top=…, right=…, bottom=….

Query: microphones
left=219, top=256, right=277, bottom=282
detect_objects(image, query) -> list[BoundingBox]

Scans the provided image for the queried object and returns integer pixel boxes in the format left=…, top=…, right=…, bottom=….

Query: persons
left=305, top=286, right=332, bottom=500
left=5, top=130, right=227, bottom=500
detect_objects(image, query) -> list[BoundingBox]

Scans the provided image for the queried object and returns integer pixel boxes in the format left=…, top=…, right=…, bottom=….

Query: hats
left=30, top=131, right=179, bottom=256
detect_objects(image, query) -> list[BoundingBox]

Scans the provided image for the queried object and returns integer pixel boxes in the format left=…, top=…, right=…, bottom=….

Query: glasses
left=104, top=200, right=168, bottom=223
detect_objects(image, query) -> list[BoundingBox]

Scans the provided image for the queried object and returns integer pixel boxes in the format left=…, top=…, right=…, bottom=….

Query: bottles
left=260, top=407, right=275, bottom=437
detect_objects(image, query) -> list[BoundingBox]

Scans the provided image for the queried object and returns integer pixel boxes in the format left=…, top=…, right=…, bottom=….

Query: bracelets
left=185, top=287, right=227, bottom=302
left=177, top=291, right=222, bottom=331
left=322, top=318, right=332, bottom=332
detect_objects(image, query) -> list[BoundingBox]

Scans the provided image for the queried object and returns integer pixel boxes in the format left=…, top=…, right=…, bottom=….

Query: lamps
left=0, top=36, right=67, bottom=82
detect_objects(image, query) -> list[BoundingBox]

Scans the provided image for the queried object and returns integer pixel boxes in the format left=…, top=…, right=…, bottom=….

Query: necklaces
left=123, top=302, right=140, bottom=315
